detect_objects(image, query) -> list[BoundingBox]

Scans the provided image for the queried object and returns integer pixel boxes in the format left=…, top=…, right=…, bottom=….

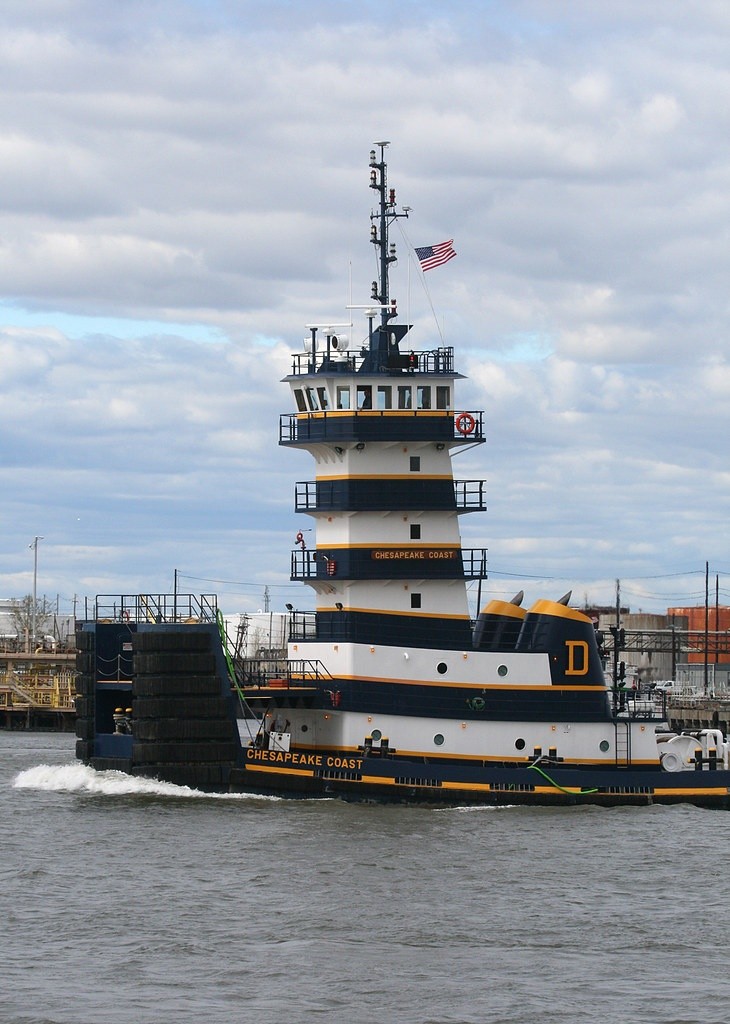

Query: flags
left=414, top=238, right=458, bottom=272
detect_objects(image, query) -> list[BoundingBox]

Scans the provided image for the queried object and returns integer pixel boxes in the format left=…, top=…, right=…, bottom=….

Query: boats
left=73, top=141, right=730, bottom=809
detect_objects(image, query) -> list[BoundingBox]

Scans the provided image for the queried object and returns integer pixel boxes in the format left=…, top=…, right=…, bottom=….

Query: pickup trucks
left=654, top=681, right=697, bottom=694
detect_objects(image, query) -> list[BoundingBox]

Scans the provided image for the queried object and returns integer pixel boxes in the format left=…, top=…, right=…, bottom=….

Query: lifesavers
left=456, top=413, right=475, bottom=435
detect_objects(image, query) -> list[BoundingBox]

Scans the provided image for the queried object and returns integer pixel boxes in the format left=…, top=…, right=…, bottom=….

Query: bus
left=0, top=635, right=56, bottom=649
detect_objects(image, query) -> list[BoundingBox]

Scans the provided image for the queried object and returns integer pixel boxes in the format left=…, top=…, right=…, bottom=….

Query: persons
left=407, top=351, right=414, bottom=372
left=41, top=635, right=56, bottom=652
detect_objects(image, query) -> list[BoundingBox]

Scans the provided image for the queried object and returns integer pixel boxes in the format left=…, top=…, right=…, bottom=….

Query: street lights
left=29, top=536, right=44, bottom=642
